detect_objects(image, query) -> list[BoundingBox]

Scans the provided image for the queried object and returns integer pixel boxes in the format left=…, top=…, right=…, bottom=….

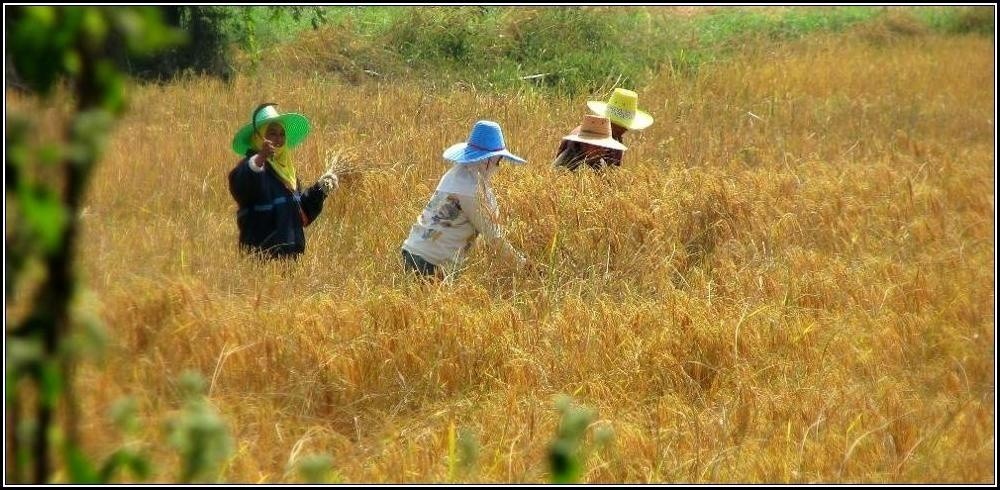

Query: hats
left=588, top=89, right=654, bottom=130
left=563, top=115, right=627, bottom=151
left=232, top=105, right=311, bottom=157
left=444, top=121, right=527, bottom=168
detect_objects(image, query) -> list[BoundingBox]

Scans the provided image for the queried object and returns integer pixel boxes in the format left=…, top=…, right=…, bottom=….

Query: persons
left=229, top=104, right=340, bottom=263
left=556, top=89, right=655, bottom=169
left=548, top=115, right=626, bottom=179
left=402, top=122, right=541, bottom=291
